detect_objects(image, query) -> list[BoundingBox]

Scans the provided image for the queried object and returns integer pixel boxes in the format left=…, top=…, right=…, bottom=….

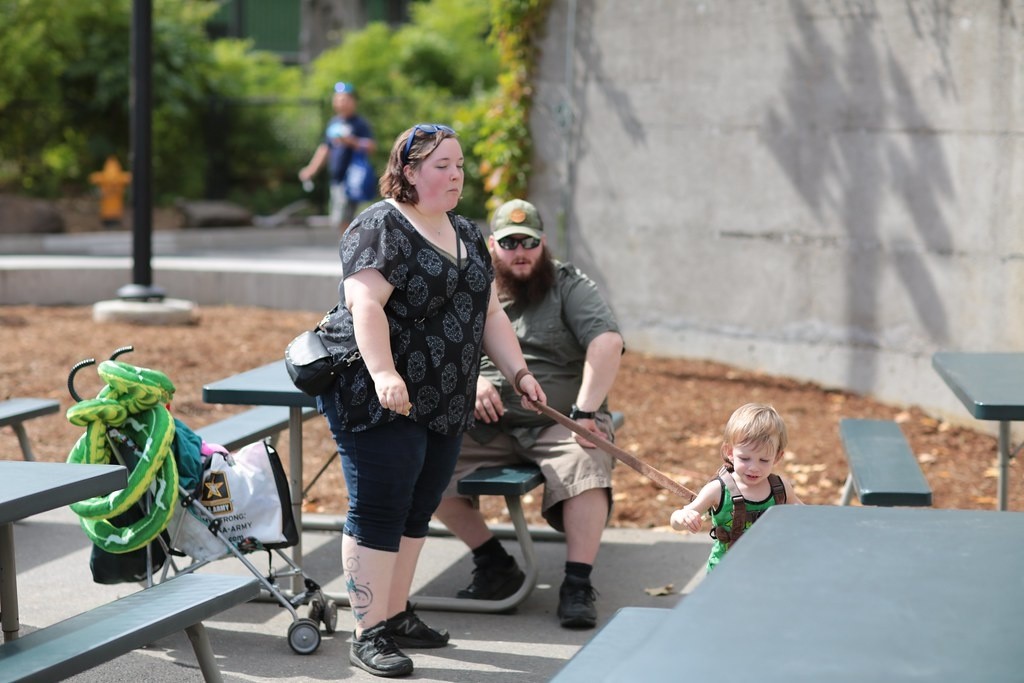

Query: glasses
left=400, top=123, right=456, bottom=162
left=333, top=82, right=354, bottom=95
left=497, top=234, right=541, bottom=252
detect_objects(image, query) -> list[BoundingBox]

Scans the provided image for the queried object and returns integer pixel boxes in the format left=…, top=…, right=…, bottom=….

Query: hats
left=491, top=198, right=545, bottom=241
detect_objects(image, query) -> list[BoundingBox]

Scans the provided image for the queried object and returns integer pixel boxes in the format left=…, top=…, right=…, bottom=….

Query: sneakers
left=377, top=601, right=453, bottom=648
left=348, top=620, right=415, bottom=679
left=452, top=554, right=527, bottom=601
left=555, top=572, right=602, bottom=629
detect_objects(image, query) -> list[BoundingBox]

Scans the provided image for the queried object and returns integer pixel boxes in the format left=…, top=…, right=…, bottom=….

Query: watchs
left=570, top=403, right=595, bottom=421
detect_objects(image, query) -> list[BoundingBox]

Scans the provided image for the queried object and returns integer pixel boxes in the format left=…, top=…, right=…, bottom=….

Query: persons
left=432, top=198, right=626, bottom=628
left=298, top=82, right=377, bottom=226
left=670, top=403, right=806, bottom=576
left=314, top=124, right=547, bottom=678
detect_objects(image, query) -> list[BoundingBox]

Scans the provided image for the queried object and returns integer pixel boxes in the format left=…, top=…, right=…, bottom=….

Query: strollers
left=67, top=345, right=338, bottom=655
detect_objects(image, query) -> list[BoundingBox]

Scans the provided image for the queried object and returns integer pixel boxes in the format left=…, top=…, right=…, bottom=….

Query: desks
left=0, top=461, right=128, bottom=642
left=203, top=362, right=318, bottom=598
left=933, top=350, right=1024, bottom=512
left=599, top=505, right=1024, bottom=683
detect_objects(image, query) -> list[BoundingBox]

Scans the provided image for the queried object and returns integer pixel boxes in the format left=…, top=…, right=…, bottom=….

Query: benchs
left=409, top=411, right=626, bottom=612
left=836, top=418, right=931, bottom=507
left=0, top=398, right=323, bottom=683
left=550, top=606, right=672, bottom=683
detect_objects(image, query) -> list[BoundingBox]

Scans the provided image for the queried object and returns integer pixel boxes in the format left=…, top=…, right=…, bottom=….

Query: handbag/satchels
left=284, top=330, right=342, bottom=397
left=343, top=147, right=378, bottom=203
left=165, top=434, right=300, bottom=560
